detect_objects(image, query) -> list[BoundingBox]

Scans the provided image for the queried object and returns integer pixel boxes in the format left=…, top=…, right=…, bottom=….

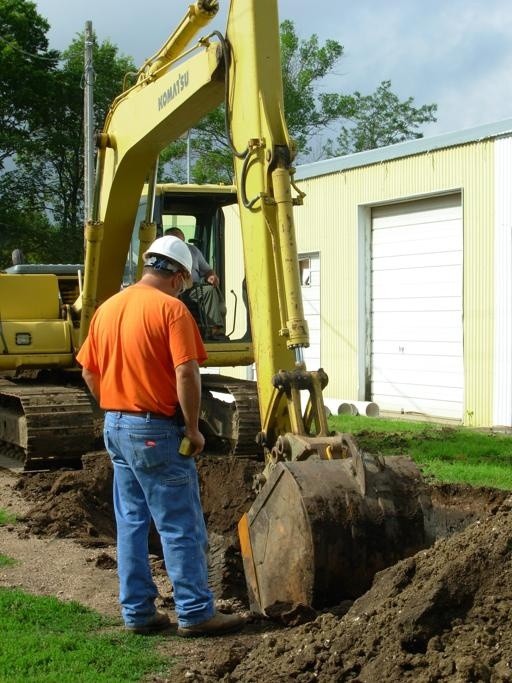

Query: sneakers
left=178, top=611, right=244, bottom=637
left=204, top=332, right=230, bottom=342
left=125, top=610, right=170, bottom=634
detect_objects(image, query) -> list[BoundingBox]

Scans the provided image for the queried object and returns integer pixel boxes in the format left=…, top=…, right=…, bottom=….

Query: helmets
left=142, top=236, right=193, bottom=289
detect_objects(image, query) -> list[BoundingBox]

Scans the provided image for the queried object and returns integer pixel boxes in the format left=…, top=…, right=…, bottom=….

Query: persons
left=75, top=234, right=243, bottom=636
left=165, top=227, right=230, bottom=342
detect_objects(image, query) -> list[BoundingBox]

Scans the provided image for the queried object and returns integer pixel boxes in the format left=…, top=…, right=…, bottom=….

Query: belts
left=108, top=410, right=174, bottom=420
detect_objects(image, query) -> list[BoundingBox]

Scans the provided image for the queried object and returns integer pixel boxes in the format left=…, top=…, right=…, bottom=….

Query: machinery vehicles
left=0, top=0, right=437, bottom=622
